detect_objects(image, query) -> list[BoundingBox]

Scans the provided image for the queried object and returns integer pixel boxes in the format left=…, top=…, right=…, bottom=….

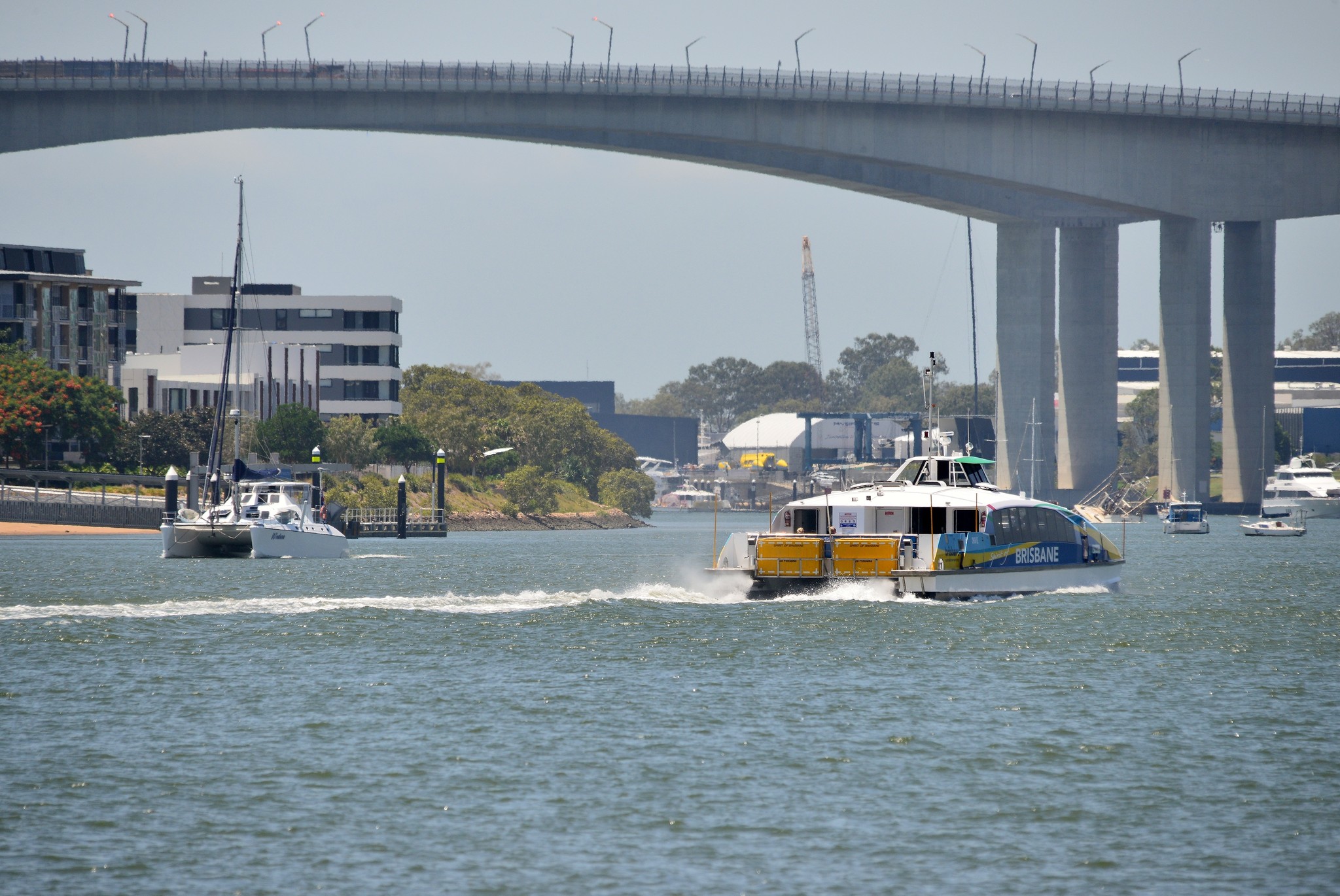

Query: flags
left=321, top=489, right=324, bottom=506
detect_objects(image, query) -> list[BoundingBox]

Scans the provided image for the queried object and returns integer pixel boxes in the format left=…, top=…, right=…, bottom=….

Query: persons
left=1007, top=508, right=1025, bottom=529
left=796, top=527, right=805, bottom=537
left=830, top=526, right=837, bottom=534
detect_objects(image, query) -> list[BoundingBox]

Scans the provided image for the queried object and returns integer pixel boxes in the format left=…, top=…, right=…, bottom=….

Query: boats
left=740, top=352, right=1128, bottom=602
left=1070, top=458, right=1159, bottom=525
left=1262, top=437, right=1340, bottom=519
left=1163, top=500, right=1211, bottom=534
left=649, top=478, right=731, bottom=513
left=701, top=530, right=761, bottom=588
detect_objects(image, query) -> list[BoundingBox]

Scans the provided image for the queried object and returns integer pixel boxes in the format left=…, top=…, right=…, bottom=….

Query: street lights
left=756, top=413, right=764, bottom=474
left=41, top=423, right=53, bottom=487
left=139, top=434, right=151, bottom=476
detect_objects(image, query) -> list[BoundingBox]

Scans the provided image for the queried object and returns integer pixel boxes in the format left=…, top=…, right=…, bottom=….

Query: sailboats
left=1154, top=404, right=1184, bottom=520
left=1238, top=405, right=1308, bottom=537
left=160, top=175, right=354, bottom=561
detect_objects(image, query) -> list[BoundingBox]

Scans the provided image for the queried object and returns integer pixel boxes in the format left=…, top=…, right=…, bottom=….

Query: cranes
left=802, top=234, right=825, bottom=408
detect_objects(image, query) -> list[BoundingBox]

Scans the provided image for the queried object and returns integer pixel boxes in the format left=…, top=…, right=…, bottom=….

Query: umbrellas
left=694, top=489, right=716, bottom=502
left=674, top=490, right=695, bottom=504
